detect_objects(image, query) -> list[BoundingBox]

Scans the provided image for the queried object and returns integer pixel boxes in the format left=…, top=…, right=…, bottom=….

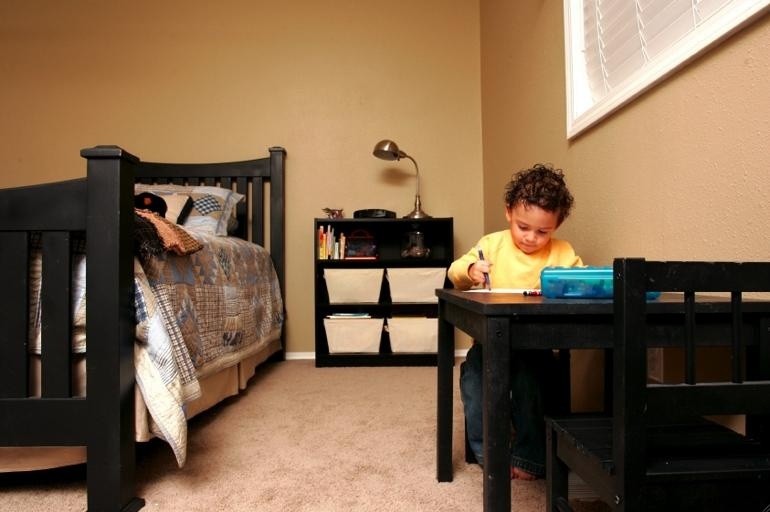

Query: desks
left=434, top=288, right=767, bottom=511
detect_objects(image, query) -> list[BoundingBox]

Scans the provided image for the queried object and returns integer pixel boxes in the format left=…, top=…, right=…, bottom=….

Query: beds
left=1, top=144, right=286, bottom=511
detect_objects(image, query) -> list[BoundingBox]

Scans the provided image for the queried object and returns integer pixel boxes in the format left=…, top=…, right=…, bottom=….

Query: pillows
left=134, top=191, right=194, bottom=227
left=133, top=182, right=246, bottom=240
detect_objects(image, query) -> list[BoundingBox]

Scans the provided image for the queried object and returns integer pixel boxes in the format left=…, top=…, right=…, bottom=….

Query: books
left=317, top=223, right=380, bottom=258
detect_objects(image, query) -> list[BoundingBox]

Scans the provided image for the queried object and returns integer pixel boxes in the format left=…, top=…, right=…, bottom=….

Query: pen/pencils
left=479, top=250, right=491, bottom=291
left=523, top=291, right=541, bottom=296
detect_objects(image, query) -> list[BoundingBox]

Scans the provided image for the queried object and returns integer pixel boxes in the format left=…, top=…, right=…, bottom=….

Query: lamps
left=372, top=140, right=433, bottom=219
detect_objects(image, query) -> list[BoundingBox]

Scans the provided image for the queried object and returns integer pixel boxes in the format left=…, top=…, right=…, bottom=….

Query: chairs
left=542, top=257, right=770, bottom=511
left=459, top=360, right=479, bottom=464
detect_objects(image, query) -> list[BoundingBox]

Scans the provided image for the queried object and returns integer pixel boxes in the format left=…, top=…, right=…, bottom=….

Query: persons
left=445, top=160, right=587, bottom=482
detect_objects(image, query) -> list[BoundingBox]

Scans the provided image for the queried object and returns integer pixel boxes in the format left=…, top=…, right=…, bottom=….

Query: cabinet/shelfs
left=313, top=217, right=454, bottom=368
